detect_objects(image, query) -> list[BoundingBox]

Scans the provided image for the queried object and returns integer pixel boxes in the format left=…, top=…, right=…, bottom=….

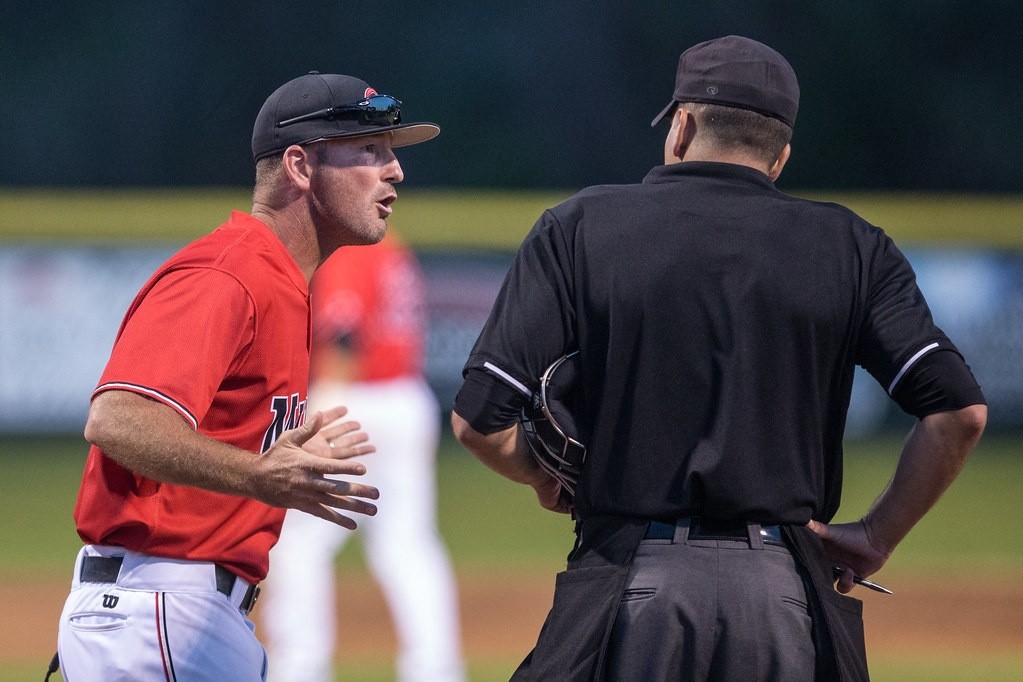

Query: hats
left=251, top=71, right=441, bottom=165
left=651, top=35, right=801, bottom=132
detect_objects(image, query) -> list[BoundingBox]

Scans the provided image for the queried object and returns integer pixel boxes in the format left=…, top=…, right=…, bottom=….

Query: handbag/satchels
left=509, top=542, right=636, bottom=682
left=792, top=528, right=870, bottom=682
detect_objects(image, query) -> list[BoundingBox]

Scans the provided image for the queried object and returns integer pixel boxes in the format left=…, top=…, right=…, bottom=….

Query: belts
left=80, top=555, right=263, bottom=616
left=642, top=519, right=787, bottom=547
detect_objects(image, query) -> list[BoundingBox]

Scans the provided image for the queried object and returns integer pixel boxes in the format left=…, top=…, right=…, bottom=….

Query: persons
left=57, top=72, right=439, bottom=682
left=264, top=226, right=461, bottom=682
left=451, top=35, right=987, bottom=682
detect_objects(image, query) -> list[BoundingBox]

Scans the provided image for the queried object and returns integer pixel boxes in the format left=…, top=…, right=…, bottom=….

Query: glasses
left=275, top=95, right=404, bottom=126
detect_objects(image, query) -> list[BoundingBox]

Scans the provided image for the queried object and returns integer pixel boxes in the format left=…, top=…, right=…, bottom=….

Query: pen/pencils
left=832, top=565, right=892, bottom=595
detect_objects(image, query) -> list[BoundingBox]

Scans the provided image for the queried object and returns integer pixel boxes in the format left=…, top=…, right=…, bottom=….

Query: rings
left=326, top=439, right=334, bottom=449
